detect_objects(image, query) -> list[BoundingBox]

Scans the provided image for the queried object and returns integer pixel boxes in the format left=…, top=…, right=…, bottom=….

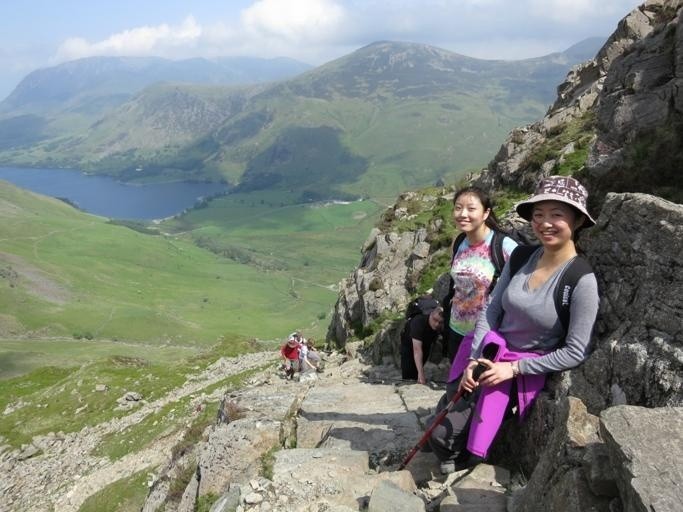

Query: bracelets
left=512, top=360, right=520, bottom=378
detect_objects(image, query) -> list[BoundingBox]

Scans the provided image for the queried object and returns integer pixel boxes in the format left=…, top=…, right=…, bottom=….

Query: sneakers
left=440, top=460, right=456, bottom=474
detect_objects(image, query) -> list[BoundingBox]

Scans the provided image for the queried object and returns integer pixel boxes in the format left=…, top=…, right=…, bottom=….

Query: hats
left=516, top=175, right=597, bottom=230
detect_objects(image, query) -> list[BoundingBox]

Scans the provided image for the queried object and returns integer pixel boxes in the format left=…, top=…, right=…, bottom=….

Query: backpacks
left=453, top=229, right=534, bottom=279
left=405, top=295, right=441, bottom=321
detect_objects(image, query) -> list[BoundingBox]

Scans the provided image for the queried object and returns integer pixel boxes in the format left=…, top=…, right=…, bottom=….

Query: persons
left=288, top=328, right=308, bottom=350
left=439, top=183, right=520, bottom=365
left=424, top=173, right=603, bottom=477
left=398, top=306, right=443, bottom=385
left=299, top=338, right=324, bottom=374
left=281, top=339, right=302, bottom=381
